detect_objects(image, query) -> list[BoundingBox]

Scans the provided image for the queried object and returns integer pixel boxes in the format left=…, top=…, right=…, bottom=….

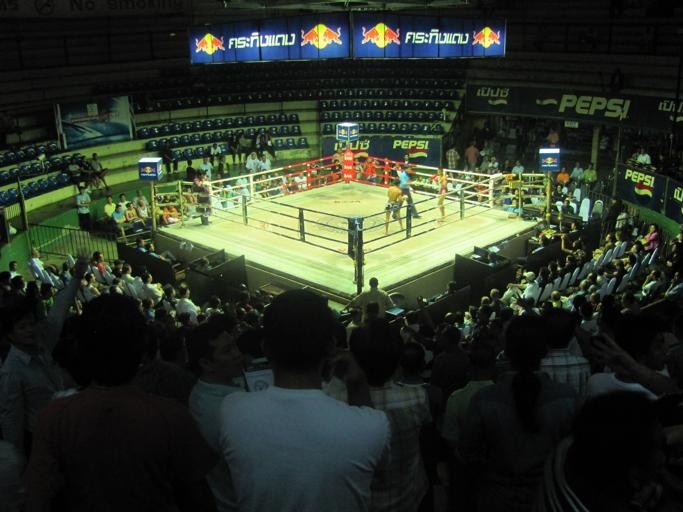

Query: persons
left=273, top=144, right=447, bottom=234
left=68, top=129, right=275, bottom=267
left=0, top=250, right=683, bottom=512
left=445, top=117, right=682, bottom=260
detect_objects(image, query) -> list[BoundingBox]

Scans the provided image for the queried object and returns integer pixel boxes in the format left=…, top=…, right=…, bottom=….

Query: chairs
left=0, top=60, right=469, bottom=209
left=479, top=241, right=682, bottom=336
